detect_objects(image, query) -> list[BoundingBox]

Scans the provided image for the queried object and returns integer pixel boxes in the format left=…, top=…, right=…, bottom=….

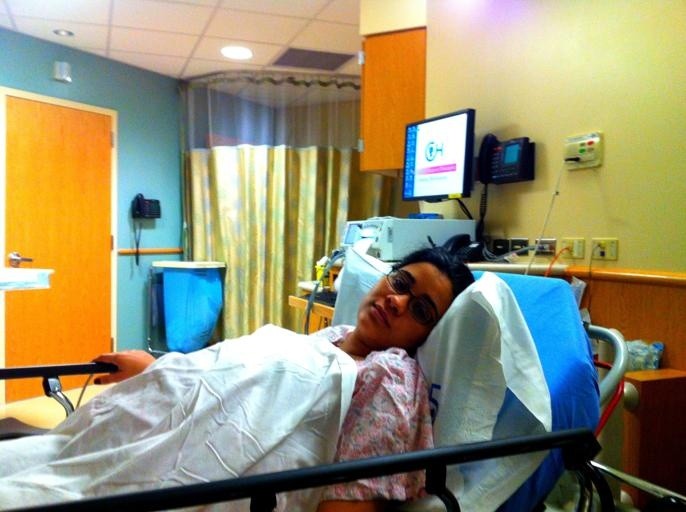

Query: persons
left=0, top=246, right=476, bottom=512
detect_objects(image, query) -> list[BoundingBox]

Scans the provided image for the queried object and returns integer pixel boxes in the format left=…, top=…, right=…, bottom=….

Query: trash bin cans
left=150, top=262, right=226, bottom=353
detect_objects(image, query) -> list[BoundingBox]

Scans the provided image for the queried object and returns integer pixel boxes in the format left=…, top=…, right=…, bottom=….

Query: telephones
left=476, top=134, right=534, bottom=185
left=444, top=234, right=483, bottom=262
left=132, top=194, right=160, bottom=218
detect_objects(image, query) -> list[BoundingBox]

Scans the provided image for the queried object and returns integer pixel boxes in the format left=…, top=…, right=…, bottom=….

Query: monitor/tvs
left=402, top=108, right=476, bottom=203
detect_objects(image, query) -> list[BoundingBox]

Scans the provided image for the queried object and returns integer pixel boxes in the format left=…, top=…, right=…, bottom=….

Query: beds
left=0, top=267, right=624, bottom=512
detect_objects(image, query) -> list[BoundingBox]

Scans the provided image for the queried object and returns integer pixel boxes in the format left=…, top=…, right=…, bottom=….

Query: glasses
left=385, top=268, right=436, bottom=326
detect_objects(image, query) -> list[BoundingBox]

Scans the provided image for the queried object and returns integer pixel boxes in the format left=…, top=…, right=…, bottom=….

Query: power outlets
left=497, top=235, right=620, bottom=262
left=562, top=137, right=602, bottom=171
